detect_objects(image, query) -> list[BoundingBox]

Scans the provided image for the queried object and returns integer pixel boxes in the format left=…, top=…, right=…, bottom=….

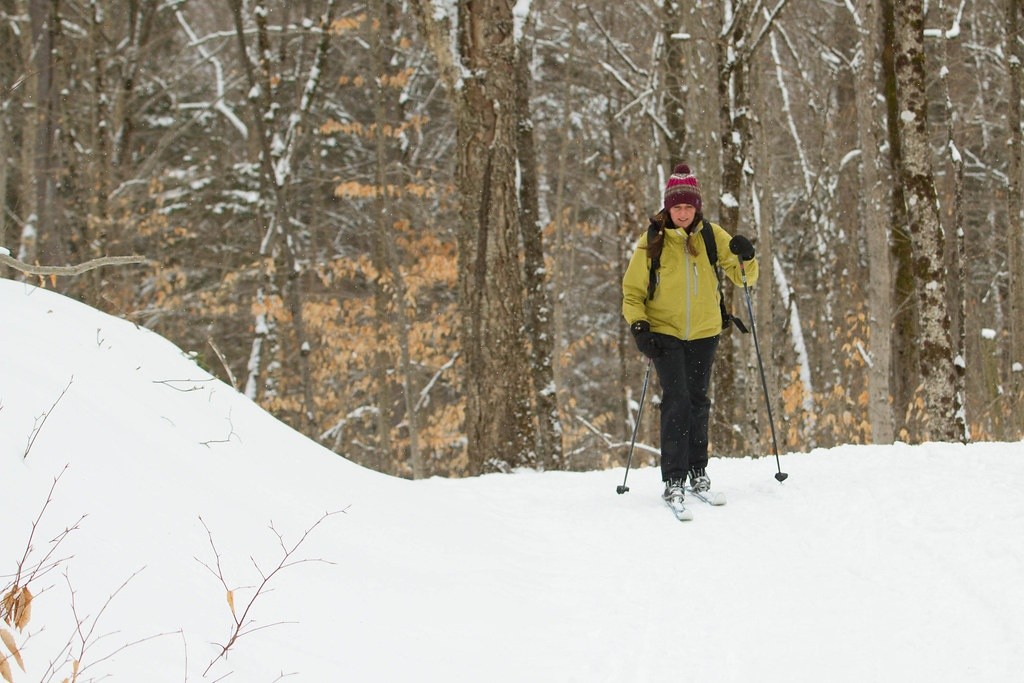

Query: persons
left=622, top=163, right=759, bottom=503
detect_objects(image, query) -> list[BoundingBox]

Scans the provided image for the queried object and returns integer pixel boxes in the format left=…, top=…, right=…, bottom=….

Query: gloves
left=729, top=235, right=755, bottom=261
left=631, top=320, right=654, bottom=357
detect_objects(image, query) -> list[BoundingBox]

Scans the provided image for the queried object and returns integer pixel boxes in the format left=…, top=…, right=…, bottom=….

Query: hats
left=664, top=164, right=701, bottom=210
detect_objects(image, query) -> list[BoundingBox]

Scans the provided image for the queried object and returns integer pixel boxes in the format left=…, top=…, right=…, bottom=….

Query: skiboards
left=660, top=484, right=726, bottom=522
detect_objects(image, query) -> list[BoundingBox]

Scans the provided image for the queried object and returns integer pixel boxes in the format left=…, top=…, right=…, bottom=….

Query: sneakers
left=688, top=467, right=711, bottom=489
left=664, top=477, right=685, bottom=500
